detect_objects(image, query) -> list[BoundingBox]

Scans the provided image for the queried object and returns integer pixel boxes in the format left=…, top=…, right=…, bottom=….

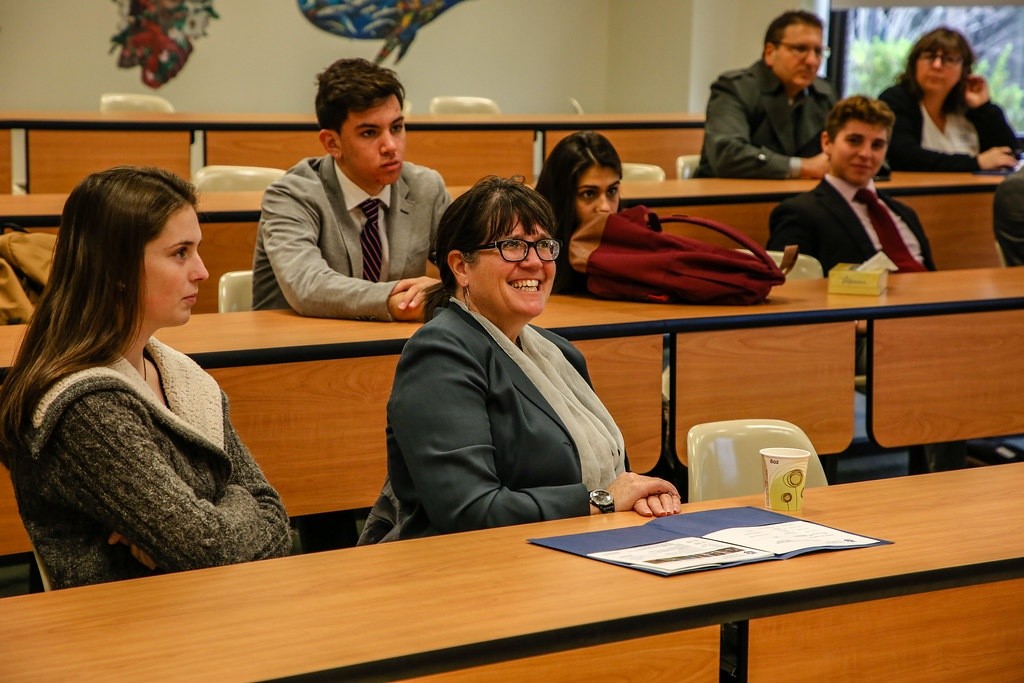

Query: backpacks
left=567, top=205, right=801, bottom=306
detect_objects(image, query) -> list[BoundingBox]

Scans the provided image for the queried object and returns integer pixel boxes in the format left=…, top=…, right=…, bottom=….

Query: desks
left=0, top=171, right=1005, bottom=314
left=0, top=460, right=1024, bottom=683
left=0, top=268, right=1024, bottom=595
left=0, top=113, right=707, bottom=195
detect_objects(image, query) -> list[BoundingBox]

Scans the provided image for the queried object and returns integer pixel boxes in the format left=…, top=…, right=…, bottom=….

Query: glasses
left=920, top=53, right=962, bottom=66
left=463, top=238, right=563, bottom=262
left=775, top=39, right=831, bottom=60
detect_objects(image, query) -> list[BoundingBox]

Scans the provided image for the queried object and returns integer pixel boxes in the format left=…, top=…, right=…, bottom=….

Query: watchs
left=588, top=489, right=616, bottom=514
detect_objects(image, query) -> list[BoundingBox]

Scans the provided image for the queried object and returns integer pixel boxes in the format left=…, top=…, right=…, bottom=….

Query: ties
left=357, top=199, right=383, bottom=284
left=853, top=188, right=928, bottom=273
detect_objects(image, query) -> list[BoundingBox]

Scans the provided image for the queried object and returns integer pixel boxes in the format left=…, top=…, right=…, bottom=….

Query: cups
left=759, top=447, right=811, bottom=518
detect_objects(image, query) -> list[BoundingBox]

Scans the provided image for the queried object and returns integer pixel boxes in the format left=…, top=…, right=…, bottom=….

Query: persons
left=534, top=128, right=624, bottom=294
left=0, top=164, right=297, bottom=592
left=383, top=171, right=684, bottom=532
left=689, top=10, right=892, bottom=182
left=767, top=94, right=939, bottom=280
left=876, top=24, right=1024, bottom=174
left=252, top=57, right=454, bottom=324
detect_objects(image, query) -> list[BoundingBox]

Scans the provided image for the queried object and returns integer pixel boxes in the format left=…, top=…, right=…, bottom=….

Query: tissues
left=828, top=250, right=899, bottom=296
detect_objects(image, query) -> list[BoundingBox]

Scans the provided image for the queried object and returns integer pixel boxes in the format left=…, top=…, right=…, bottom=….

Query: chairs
left=621, top=162, right=666, bottom=182
left=429, top=95, right=502, bottom=115
left=687, top=418, right=829, bottom=503
left=193, top=164, right=289, bottom=192
left=99, top=91, right=175, bottom=115
